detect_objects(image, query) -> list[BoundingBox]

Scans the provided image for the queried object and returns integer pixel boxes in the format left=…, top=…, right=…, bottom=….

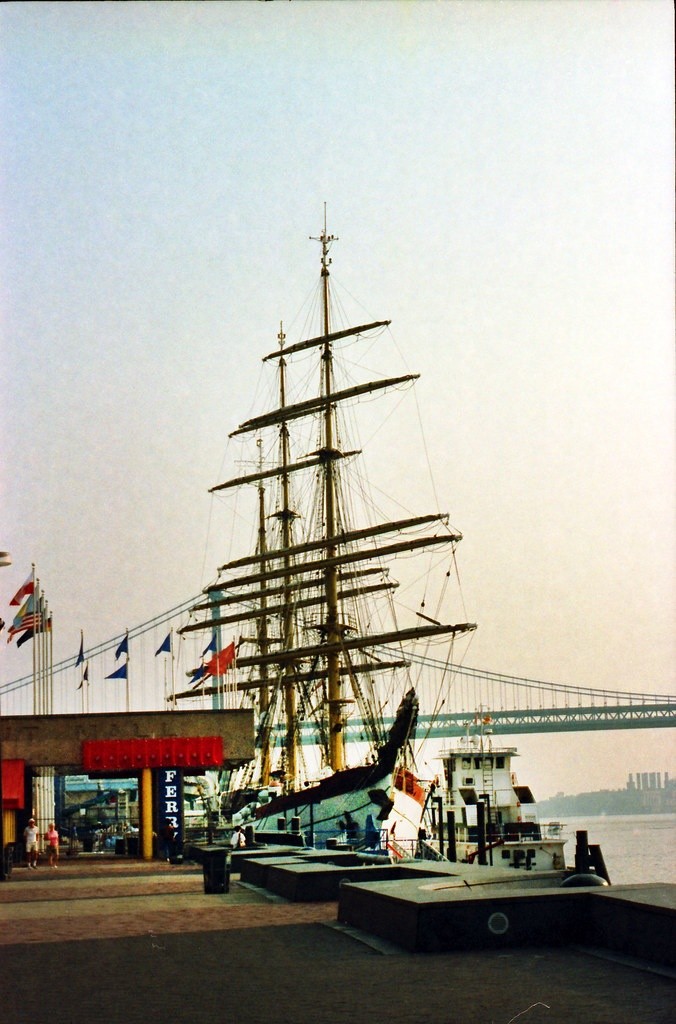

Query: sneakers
left=27, top=866, right=38, bottom=870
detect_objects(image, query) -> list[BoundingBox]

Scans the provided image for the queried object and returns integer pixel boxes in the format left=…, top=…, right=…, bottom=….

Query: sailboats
left=182, top=195, right=481, bottom=854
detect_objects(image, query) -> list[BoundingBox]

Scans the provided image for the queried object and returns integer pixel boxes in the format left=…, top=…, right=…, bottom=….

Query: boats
left=418, top=703, right=568, bottom=879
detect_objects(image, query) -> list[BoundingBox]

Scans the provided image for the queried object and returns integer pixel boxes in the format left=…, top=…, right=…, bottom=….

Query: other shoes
left=51, top=863, right=57, bottom=869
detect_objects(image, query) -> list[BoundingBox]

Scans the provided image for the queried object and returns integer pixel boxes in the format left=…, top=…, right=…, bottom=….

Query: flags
left=205, top=643, right=240, bottom=677
left=6, top=569, right=52, bottom=646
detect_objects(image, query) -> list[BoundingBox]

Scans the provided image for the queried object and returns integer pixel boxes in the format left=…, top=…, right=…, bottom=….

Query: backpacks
left=236, top=832, right=246, bottom=850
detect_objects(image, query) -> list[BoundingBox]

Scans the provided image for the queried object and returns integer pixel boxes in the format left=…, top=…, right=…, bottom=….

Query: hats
left=28, top=817, right=34, bottom=821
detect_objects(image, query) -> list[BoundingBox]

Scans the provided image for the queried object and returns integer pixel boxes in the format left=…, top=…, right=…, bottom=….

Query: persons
left=70, top=812, right=137, bottom=856
left=230, top=825, right=247, bottom=850
left=161, top=818, right=178, bottom=862
left=24, top=817, right=42, bottom=873
left=42, top=823, right=62, bottom=870
